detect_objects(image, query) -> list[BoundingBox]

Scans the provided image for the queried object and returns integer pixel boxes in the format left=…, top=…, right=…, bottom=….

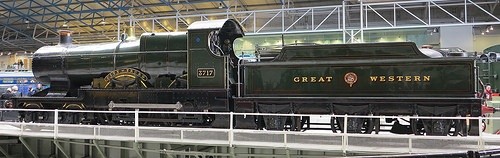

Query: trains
left=0, top=16, right=500, bottom=136
left=1, top=59, right=52, bottom=99
